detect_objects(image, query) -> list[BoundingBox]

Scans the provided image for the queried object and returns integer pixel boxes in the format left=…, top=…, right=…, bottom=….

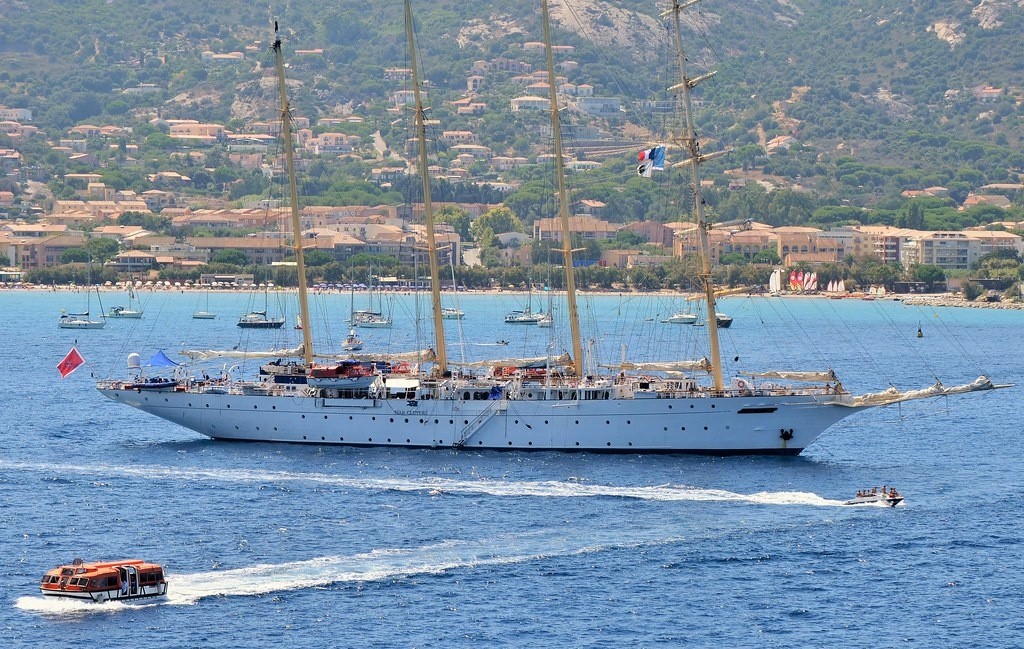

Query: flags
left=637, top=145, right=666, bottom=171
left=56, top=345, right=86, bottom=379
left=637, top=158, right=653, bottom=178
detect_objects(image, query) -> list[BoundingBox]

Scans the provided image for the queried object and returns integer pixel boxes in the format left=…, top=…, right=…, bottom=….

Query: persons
left=855, top=484, right=899, bottom=500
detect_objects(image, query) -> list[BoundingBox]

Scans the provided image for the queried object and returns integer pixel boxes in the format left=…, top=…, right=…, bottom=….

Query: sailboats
left=88, top=1, right=1023, bottom=456
left=237, top=243, right=285, bottom=328
left=105, top=256, right=144, bottom=319
left=58, top=253, right=107, bottom=329
left=353, top=261, right=382, bottom=318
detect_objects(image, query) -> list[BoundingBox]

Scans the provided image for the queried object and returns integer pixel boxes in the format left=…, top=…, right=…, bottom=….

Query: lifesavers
left=738, top=381, right=744, bottom=388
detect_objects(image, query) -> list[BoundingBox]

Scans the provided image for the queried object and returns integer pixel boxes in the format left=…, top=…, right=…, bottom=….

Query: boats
left=351, top=318, right=392, bottom=328
left=712, top=312, right=732, bottom=328
left=40, top=559, right=167, bottom=606
left=440, top=306, right=465, bottom=321
left=845, top=494, right=905, bottom=510
left=537, top=318, right=554, bottom=328
left=502, top=311, right=546, bottom=325
left=193, top=278, right=218, bottom=320
left=661, top=313, right=699, bottom=325
